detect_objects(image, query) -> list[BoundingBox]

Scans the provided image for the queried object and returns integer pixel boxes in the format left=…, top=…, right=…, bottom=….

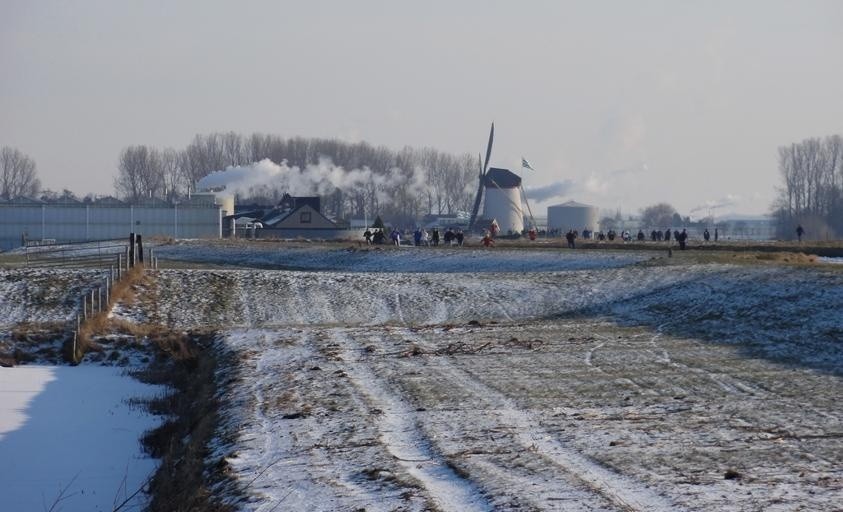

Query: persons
left=703, top=228, right=710, bottom=241
left=713, top=227, right=719, bottom=241
left=360, top=221, right=500, bottom=248
left=564, top=229, right=576, bottom=250
left=678, top=228, right=687, bottom=249
left=795, top=224, right=805, bottom=241
left=572, top=227, right=680, bottom=242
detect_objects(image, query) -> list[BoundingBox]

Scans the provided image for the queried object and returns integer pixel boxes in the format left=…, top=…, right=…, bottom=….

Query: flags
left=521, top=158, right=534, bottom=172
